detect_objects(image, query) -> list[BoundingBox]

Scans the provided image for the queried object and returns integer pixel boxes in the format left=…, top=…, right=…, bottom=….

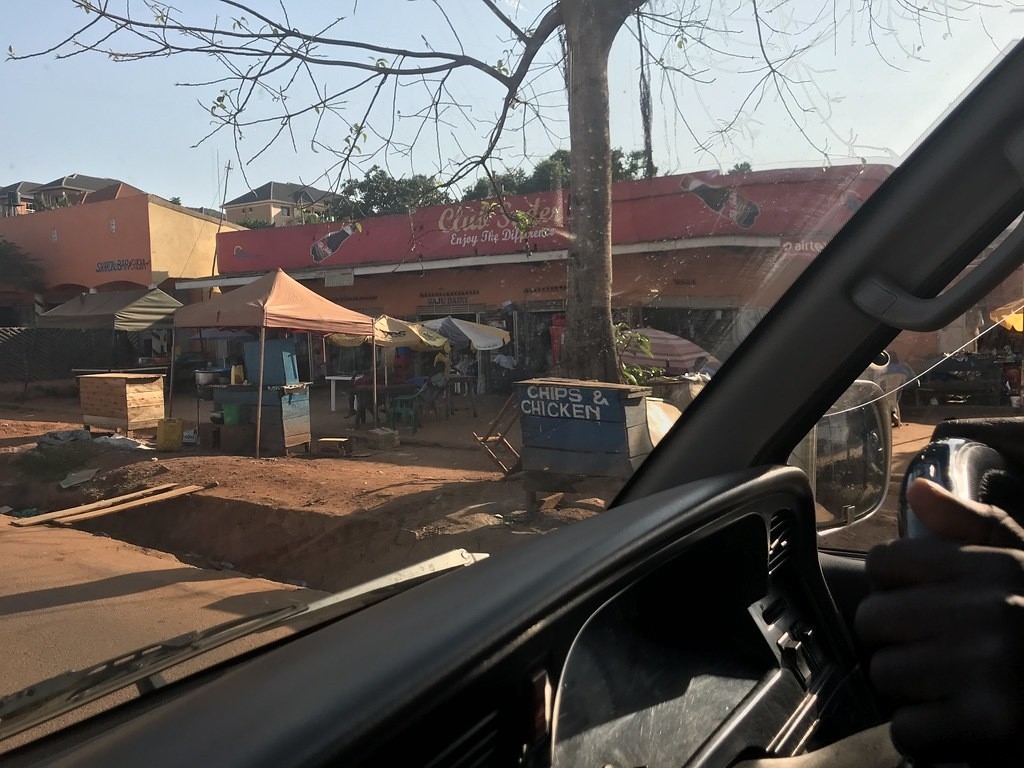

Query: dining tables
left=412, top=373, right=479, bottom=421
left=323, top=375, right=367, bottom=414
left=354, top=382, right=417, bottom=423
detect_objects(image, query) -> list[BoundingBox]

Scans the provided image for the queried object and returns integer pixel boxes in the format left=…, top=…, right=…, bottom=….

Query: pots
left=196, top=370, right=221, bottom=386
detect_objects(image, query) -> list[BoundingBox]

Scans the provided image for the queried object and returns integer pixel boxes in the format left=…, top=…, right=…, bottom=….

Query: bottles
left=156, top=418, right=183, bottom=453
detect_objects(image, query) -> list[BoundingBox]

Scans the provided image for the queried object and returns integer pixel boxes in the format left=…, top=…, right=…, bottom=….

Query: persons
left=853, top=477, right=1024, bottom=768
left=691, top=356, right=716, bottom=380
left=490, top=345, right=526, bottom=383
left=875, top=350, right=910, bottom=427
left=432, top=347, right=463, bottom=415
left=343, top=342, right=381, bottom=419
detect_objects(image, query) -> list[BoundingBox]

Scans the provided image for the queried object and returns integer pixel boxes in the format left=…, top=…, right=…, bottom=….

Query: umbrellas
left=414, top=315, right=511, bottom=401
left=989, top=297, right=1024, bottom=333
left=187, top=326, right=254, bottom=368
left=615, top=325, right=723, bottom=376
left=329, top=313, right=452, bottom=386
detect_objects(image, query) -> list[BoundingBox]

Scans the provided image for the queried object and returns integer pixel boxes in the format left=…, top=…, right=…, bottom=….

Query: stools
left=389, top=407, right=420, bottom=432
left=394, top=395, right=419, bottom=420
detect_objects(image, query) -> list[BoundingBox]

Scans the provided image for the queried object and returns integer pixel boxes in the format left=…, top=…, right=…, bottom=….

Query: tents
left=168, top=266, right=379, bottom=460
left=21, top=288, right=184, bottom=406
